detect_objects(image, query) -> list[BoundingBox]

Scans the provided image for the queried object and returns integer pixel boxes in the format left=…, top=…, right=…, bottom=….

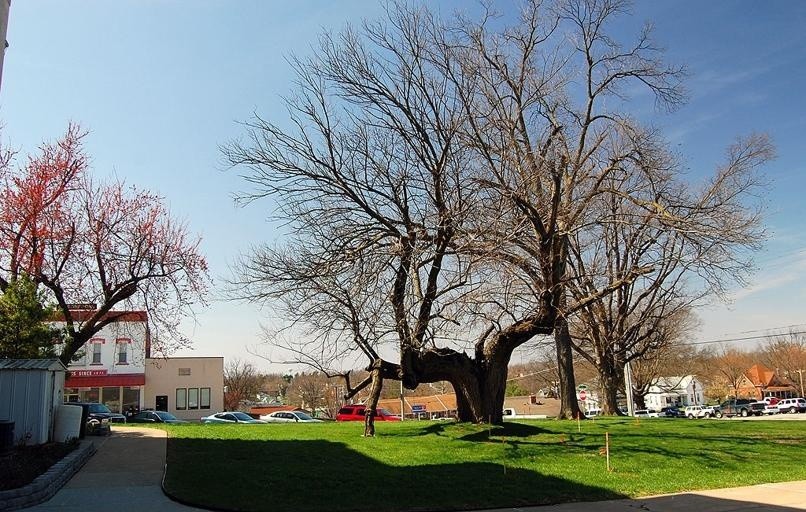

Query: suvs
left=336, top=404, right=401, bottom=421
left=67, top=402, right=127, bottom=424
left=661, top=406, right=720, bottom=419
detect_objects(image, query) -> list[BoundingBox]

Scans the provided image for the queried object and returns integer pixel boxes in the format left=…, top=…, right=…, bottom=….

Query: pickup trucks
left=714, top=398, right=780, bottom=417
left=635, top=410, right=659, bottom=418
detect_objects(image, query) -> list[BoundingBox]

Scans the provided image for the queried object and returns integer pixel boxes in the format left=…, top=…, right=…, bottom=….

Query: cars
left=132, top=411, right=188, bottom=424
left=260, top=410, right=323, bottom=423
left=200, top=412, right=269, bottom=424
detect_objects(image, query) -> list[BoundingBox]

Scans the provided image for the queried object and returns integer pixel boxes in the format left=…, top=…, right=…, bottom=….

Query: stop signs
left=579, top=391, right=586, bottom=400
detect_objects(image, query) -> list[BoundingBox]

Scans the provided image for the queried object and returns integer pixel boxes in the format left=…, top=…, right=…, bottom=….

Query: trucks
left=501, top=408, right=548, bottom=420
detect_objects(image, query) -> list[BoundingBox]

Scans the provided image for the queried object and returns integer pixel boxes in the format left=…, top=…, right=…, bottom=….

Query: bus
left=584, top=409, right=602, bottom=417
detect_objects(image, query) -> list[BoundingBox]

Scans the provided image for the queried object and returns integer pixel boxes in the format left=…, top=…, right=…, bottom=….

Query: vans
left=776, top=398, right=806, bottom=414
